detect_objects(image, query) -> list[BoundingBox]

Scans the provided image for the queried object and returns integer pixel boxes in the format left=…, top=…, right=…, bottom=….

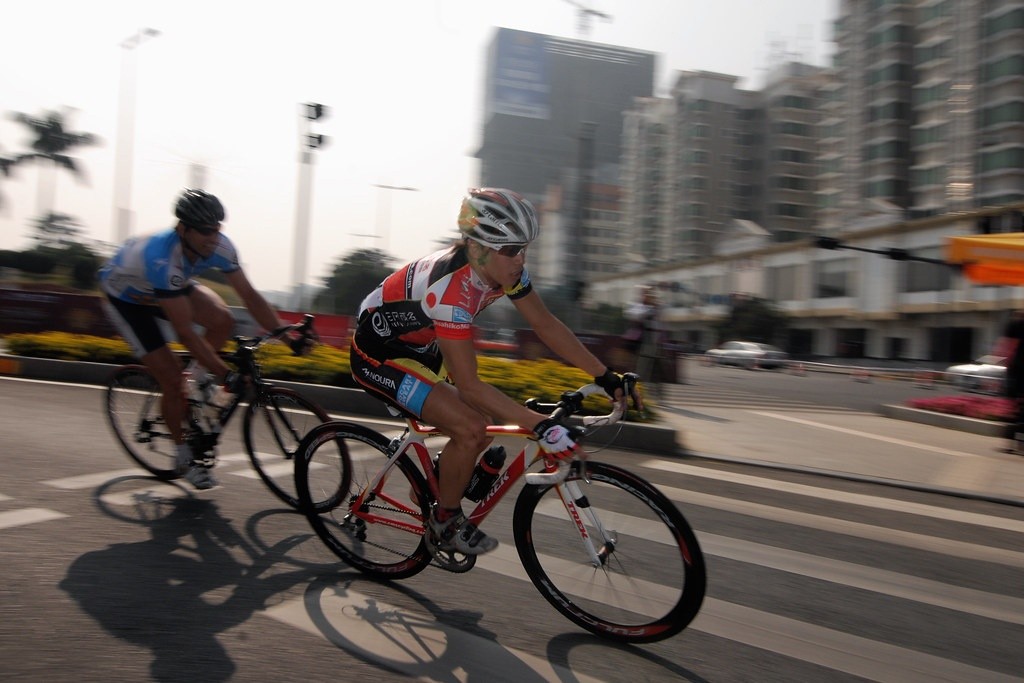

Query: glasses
left=461, top=230, right=528, bottom=257
left=179, top=220, right=221, bottom=237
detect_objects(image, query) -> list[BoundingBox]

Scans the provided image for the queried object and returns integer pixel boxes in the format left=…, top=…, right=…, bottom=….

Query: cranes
left=562, top=1, right=613, bottom=40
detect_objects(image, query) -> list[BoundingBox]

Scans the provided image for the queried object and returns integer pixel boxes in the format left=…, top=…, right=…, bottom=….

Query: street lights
left=106, top=25, right=163, bottom=251
left=289, top=101, right=324, bottom=312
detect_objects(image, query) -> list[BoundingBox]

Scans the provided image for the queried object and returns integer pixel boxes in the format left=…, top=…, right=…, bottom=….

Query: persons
left=101, top=191, right=317, bottom=488
left=350, top=188, right=644, bottom=555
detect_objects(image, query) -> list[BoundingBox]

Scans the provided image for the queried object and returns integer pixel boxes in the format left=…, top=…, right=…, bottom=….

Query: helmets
left=175, top=188, right=224, bottom=224
left=458, top=187, right=539, bottom=242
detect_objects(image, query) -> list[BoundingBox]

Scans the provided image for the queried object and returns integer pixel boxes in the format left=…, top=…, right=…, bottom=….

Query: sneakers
left=428, top=509, right=498, bottom=555
left=184, top=369, right=213, bottom=403
left=409, top=487, right=419, bottom=509
left=180, top=457, right=211, bottom=488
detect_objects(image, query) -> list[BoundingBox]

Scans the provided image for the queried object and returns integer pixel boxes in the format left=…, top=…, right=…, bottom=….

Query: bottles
left=464, top=445, right=507, bottom=503
left=408, top=450, right=443, bottom=505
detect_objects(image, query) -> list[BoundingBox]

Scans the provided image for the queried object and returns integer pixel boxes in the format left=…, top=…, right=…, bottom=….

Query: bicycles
left=292, top=370, right=706, bottom=644
left=100, top=313, right=354, bottom=515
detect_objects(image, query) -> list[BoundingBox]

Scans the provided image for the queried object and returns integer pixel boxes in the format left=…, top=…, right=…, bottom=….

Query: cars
left=944, top=361, right=1008, bottom=393
left=704, top=338, right=791, bottom=369
